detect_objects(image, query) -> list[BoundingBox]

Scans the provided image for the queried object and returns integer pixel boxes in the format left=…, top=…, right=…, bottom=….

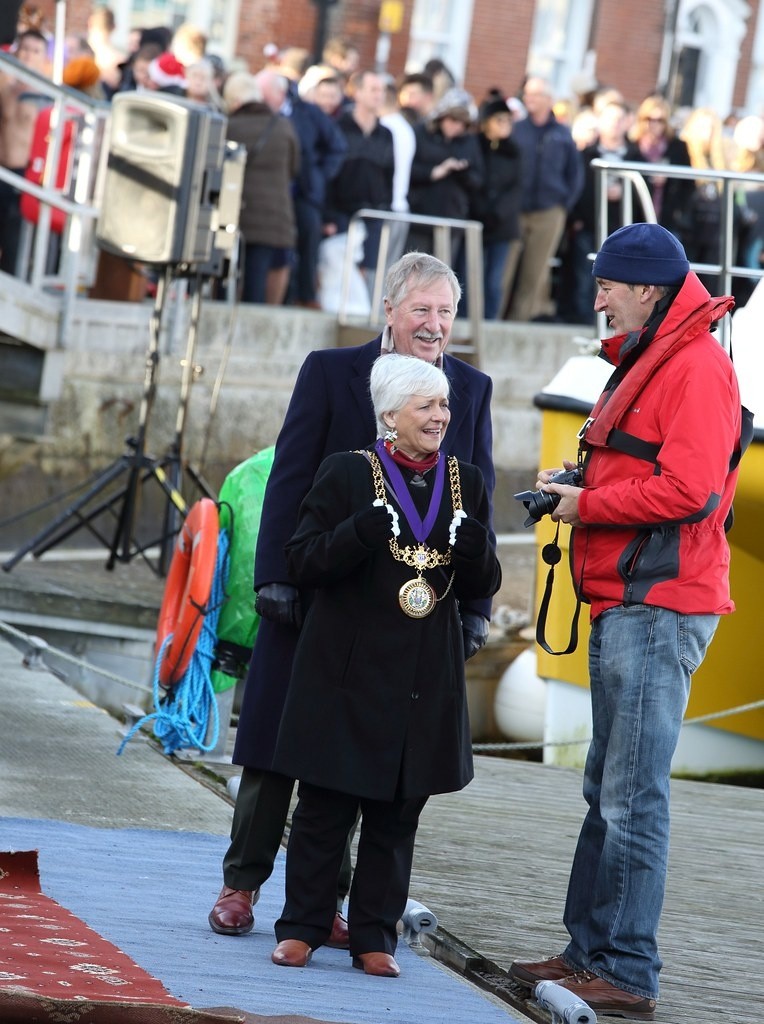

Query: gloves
left=254, top=583, right=301, bottom=629
left=449, top=510, right=489, bottom=563
left=461, top=610, right=489, bottom=661
left=356, top=499, right=401, bottom=549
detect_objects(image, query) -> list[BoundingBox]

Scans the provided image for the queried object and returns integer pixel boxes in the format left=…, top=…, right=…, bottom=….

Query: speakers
left=167, top=141, right=246, bottom=278
left=94, top=90, right=228, bottom=267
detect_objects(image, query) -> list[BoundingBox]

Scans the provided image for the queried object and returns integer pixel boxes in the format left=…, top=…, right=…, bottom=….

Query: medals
left=398, top=580, right=436, bottom=616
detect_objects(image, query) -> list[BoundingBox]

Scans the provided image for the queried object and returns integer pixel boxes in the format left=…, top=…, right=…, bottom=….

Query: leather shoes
left=531, top=969, right=656, bottom=1021
left=323, top=912, right=349, bottom=949
left=509, top=954, right=576, bottom=988
left=209, top=885, right=261, bottom=935
left=272, top=939, right=312, bottom=967
left=352, top=952, right=400, bottom=978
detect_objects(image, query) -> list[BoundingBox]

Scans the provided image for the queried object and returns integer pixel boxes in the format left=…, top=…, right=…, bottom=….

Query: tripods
left=0, top=265, right=223, bottom=578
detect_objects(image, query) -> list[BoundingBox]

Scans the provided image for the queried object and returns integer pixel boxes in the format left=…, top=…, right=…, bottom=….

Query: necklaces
left=351, top=448, right=461, bottom=569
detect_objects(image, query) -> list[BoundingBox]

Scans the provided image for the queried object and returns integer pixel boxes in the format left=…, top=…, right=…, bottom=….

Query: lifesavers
left=152, top=496, right=222, bottom=688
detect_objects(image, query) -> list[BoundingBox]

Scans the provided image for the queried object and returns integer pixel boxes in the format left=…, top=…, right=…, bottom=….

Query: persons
left=1, top=0, right=764, bottom=320
left=510, top=224, right=752, bottom=1015
left=208, top=253, right=501, bottom=977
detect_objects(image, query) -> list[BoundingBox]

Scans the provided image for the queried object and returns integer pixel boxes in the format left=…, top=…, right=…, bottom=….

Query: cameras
left=514, top=465, right=587, bottom=528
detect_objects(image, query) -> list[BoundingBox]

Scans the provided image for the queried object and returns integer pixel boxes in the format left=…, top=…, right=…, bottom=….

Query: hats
left=426, top=88, right=479, bottom=134
left=63, top=54, right=98, bottom=88
left=592, top=223, right=690, bottom=285
left=479, top=88, right=510, bottom=120
left=148, top=52, right=189, bottom=90
